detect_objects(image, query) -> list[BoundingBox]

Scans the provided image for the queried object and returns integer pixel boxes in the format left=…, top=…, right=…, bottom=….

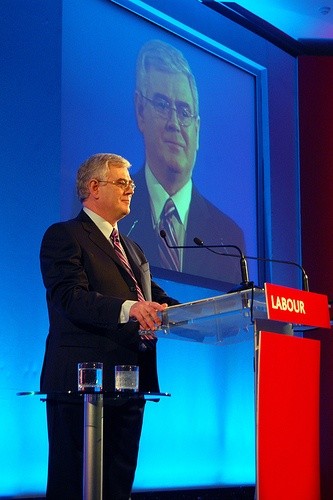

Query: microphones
left=193, top=237, right=309, bottom=292
left=160, top=230, right=262, bottom=294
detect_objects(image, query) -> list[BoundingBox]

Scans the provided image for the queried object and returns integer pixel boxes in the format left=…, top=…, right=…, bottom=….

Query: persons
left=41, top=153, right=238, bottom=500
left=117, top=42, right=248, bottom=284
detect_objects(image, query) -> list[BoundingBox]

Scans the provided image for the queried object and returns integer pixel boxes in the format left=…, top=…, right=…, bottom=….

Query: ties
left=152, top=200, right=180, bottom=272
left=111, top=228, right=153, bottom=339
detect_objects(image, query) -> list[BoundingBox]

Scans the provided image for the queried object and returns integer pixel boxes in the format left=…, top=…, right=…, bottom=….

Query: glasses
left=98, top=179, right=137, bottom=190
left=138, top=94, right=198, bottom=127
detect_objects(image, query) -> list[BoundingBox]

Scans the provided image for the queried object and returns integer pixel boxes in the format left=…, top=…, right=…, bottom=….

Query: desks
left=16, top=390, right=171, bottom=500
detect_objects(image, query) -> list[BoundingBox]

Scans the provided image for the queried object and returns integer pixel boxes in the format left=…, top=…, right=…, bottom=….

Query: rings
left=143, top=313, right=149, bottom=320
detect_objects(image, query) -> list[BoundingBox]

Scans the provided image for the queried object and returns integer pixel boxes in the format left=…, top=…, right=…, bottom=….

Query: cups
left=115, top=365, right=139, bottom=393
left=78, top=362, right=102, bottom=391
